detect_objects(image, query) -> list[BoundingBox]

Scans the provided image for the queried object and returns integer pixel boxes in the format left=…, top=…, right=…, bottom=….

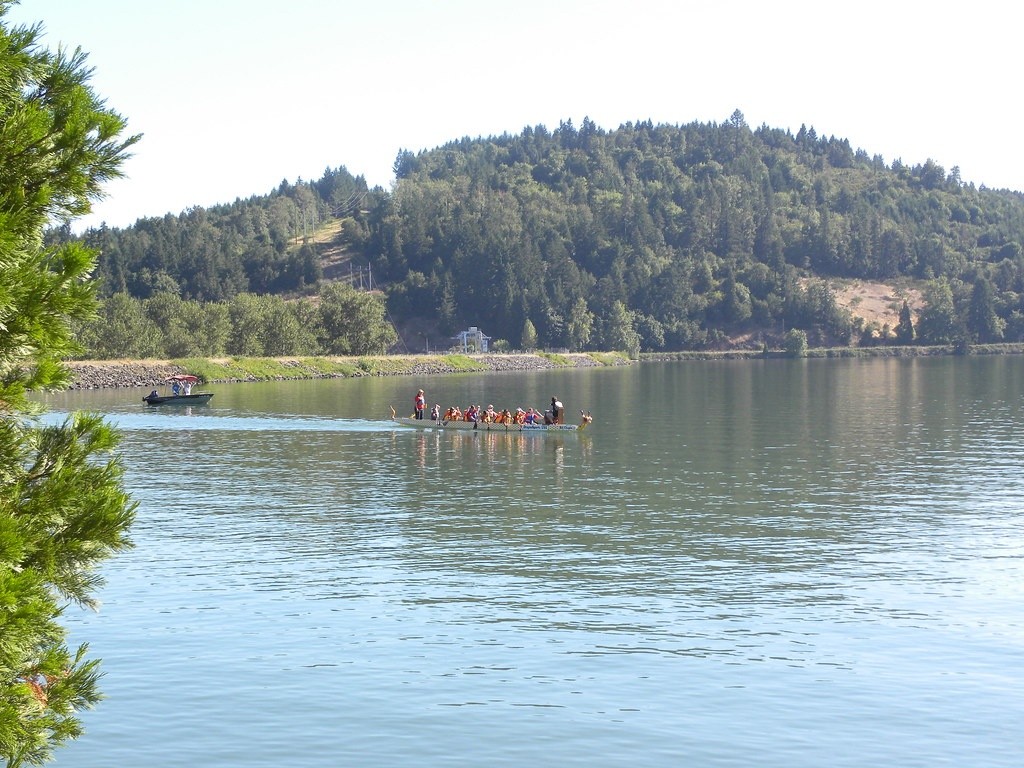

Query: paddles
left=443, top=418, right=454, bottom=426
left=472, top=407, right=480, bottom=431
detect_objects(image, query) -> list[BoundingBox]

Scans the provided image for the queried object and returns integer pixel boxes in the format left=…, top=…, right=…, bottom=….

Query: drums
left=544, top=409, right=554, bottom=424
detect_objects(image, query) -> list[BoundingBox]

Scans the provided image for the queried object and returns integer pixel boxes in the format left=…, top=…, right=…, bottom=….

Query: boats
left=389, top=405, right=594, bottom=432
left=142, top=374, right=214, bottom=406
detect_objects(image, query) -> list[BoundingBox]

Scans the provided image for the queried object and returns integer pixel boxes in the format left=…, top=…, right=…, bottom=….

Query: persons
left=443, top=407, right=463, bottom=421
left=172, top=380, right=193, bottom=396
left=497, top=406, right=540, bottom=426
left=430, top=403, right=441, bottom=419
left=545, top=395, right=565, bottom=426
left=414, top=389, right=426, bottom=419
left=464, top=404, right=499, bottom=423
